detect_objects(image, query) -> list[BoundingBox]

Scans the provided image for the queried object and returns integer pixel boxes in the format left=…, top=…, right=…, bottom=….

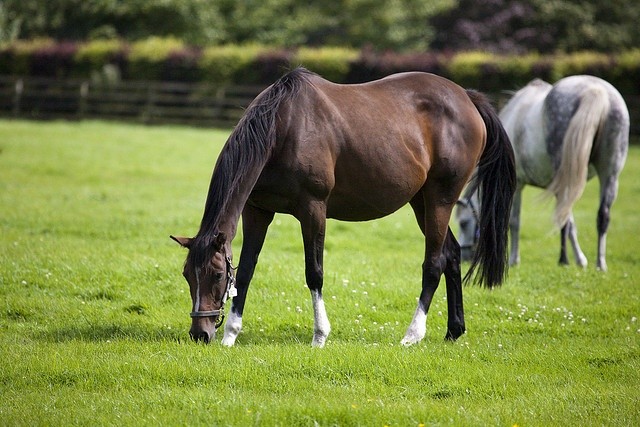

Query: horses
left=454, top=74, right=631, bottom=275
left=169, top=54, right=518, bottom=350
